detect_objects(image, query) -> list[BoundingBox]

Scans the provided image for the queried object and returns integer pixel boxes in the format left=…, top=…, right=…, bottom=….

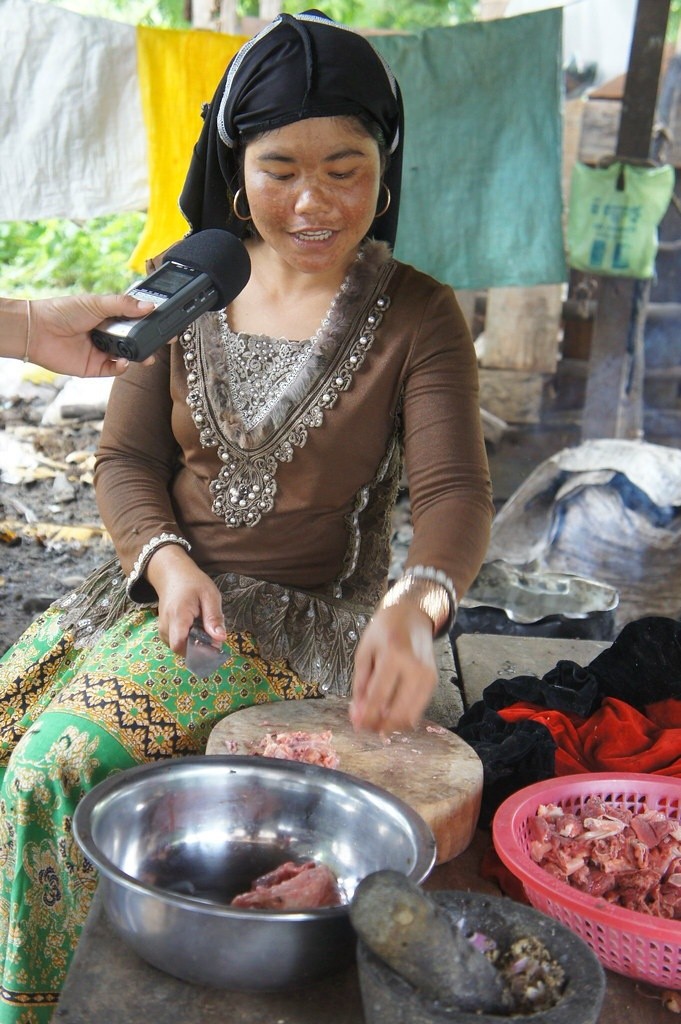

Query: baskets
left=493, top=772, right=681, bottom=990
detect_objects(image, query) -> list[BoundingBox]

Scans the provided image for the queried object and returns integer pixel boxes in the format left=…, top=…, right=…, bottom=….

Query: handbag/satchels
left=563, top=158, right=673, bottom=278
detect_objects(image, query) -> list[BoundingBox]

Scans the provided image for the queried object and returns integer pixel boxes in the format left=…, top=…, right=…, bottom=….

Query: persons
left=0, top=295, right=177, bottom=379
left=0, top=6, right=493, bottom=1024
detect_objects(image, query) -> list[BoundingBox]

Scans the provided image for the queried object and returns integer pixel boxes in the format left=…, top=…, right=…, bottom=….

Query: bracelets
left=22, top=298, right=31, bottom=362
left=377, top=575, right=451, bottom=636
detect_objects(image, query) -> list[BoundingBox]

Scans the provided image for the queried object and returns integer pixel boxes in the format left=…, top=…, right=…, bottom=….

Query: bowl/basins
left=73, top=755, right=437, bottom=988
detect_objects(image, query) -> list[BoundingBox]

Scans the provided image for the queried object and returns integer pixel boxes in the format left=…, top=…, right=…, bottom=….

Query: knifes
left=184, top=617, right=231, bottom=679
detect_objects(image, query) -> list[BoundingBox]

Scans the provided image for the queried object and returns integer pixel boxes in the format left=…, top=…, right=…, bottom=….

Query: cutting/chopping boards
left=206, top=698, right=483, bottom=865
